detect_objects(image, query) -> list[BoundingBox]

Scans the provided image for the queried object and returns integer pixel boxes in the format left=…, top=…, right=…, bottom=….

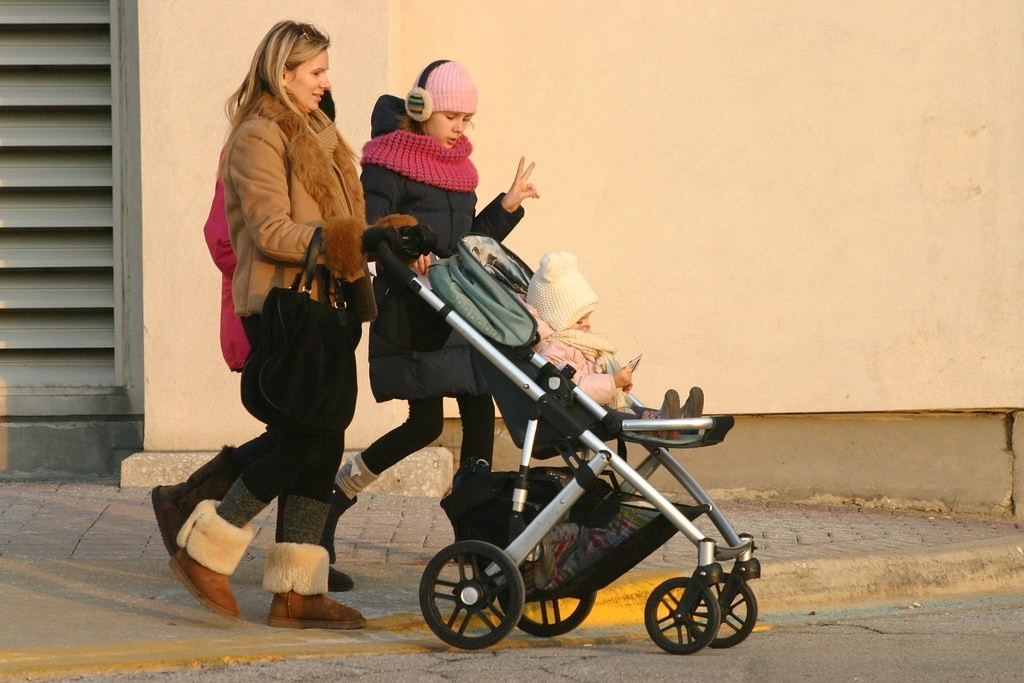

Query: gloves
left=363, top=224, right=404, bottom=256
left=398, top=222, right=437, bottom=258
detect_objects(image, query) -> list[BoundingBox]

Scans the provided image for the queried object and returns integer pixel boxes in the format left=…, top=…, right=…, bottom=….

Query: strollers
left=365, top=230, right=765, bottom=655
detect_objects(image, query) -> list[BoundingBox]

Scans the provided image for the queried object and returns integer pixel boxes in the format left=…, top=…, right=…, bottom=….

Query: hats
left=412, top=59, right=478, bottom=114
left=525, top=251, right=598, bottom=332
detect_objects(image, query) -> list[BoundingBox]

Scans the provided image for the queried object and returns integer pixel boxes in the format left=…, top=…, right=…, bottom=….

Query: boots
left=640, top=389, right=680, bottom=440
left=152, top=445, right=238, bottom=558
left=680, top=386, right=704, bottom=435
left=319, top=470, right=357, bottom=592
left=263, top=542, right=366, bottom=629
left=168, top=499, right=254, bottom=622
left=441, top=488, right=473, bottom=563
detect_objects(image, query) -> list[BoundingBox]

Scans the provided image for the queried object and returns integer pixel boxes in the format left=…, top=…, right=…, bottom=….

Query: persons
left=168, top=20, right=435, bottom=626
left=518, top=250, right=704, bottom=441
left=320, top=58, right=541, bottom=565
left=151, top=88, right=354, bottom=591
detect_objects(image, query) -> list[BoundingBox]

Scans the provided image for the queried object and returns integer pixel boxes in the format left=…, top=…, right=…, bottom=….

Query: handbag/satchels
left=240, top=226, right=358, bottom=436
